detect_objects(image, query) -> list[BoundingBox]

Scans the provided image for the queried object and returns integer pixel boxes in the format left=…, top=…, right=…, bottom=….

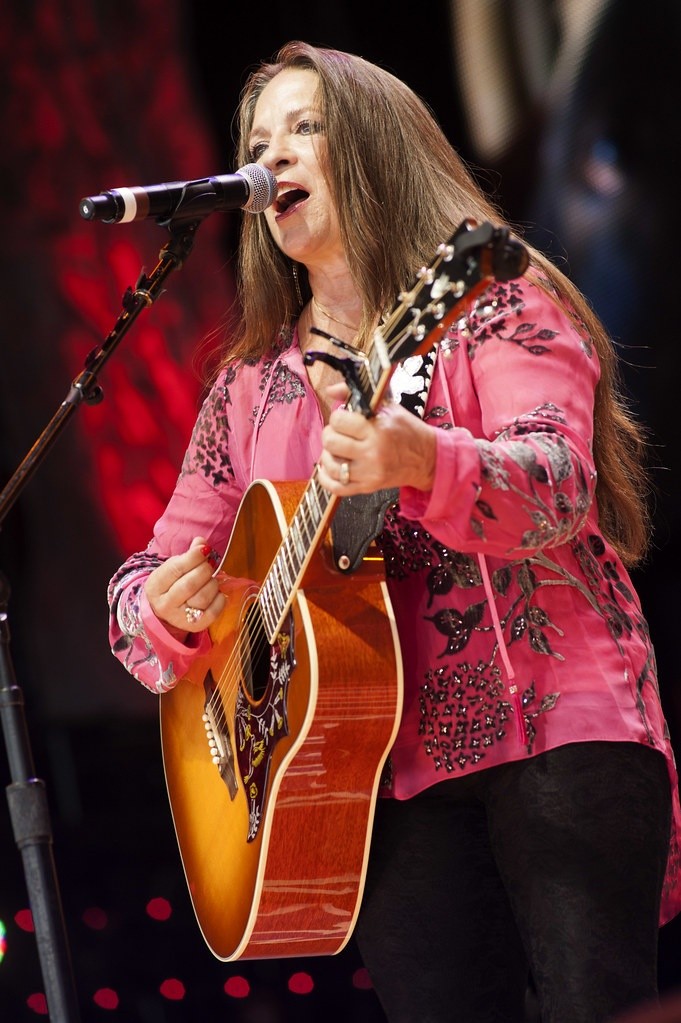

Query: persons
left=106, top=38, right=681, bottom=1023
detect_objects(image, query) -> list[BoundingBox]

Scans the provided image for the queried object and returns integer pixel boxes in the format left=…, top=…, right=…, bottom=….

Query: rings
left=340, top=460, right=350, bottom=485
left=184, top=601, right=205, bottom=626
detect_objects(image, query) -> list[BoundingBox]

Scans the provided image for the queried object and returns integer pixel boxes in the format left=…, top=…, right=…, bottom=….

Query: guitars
left=157, top=213, right=531, bottom=966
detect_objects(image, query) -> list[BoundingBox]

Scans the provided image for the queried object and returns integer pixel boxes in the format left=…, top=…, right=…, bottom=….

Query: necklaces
left=311, top=296, right=360, bottom=331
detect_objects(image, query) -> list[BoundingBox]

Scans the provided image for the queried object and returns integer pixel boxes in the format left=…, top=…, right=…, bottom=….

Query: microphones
left=78, top=163, right=278, bottom=225
